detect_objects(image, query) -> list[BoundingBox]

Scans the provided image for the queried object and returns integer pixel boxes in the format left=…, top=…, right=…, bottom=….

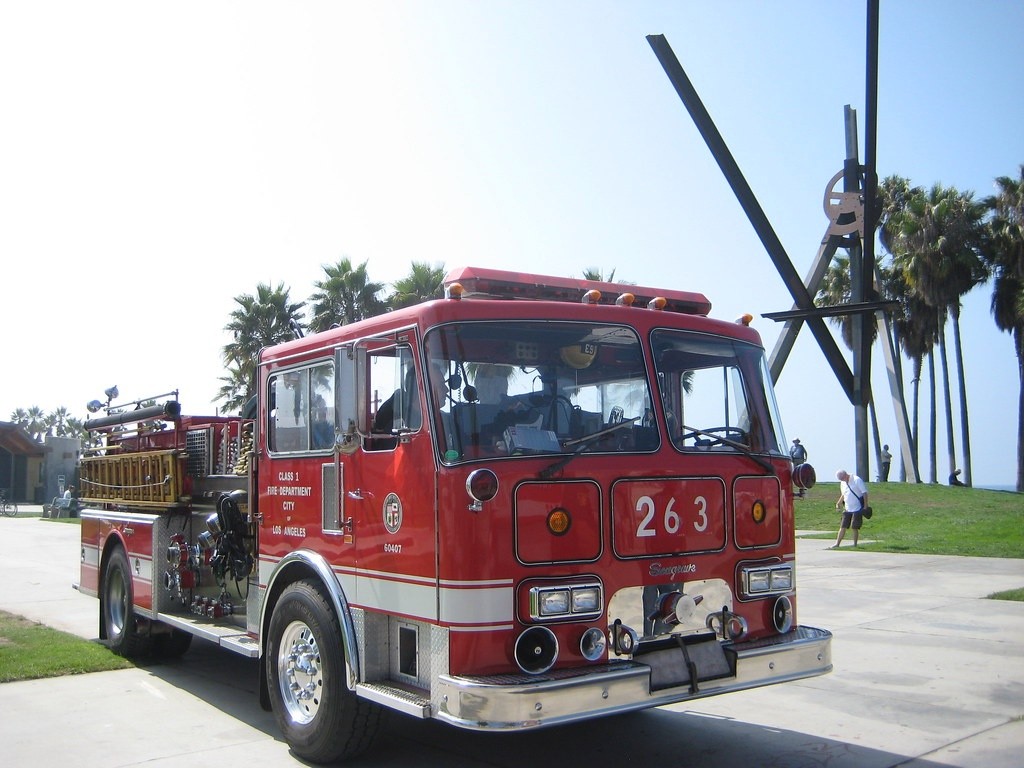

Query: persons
left=949, top=469, right=968, bottom=487
left=829, top=470, right=867, bottom=547
left=790, top=439, right=808, bottom=466
left=63, top=485, right=75, bottom=500
left=376, top=361, right=472, bottom=450
left=880, top=445, right=892, bottom=483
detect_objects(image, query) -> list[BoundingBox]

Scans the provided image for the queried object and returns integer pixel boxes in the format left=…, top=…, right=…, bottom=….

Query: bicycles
left=0, top=488, right=18, bottom=517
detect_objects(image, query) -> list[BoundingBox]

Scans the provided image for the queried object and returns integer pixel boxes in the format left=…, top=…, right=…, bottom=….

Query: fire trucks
left=71, top=267, right=834, bottom=768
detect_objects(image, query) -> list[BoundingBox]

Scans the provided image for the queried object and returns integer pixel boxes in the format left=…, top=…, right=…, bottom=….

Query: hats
left=792, top=437, right=801, bottom=443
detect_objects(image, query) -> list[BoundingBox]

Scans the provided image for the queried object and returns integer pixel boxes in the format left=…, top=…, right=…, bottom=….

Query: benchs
left=41, top=497, right=85, bottom=519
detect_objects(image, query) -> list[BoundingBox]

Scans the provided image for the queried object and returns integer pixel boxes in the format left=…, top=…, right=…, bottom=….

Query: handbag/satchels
left=858, top=496, right=873, bottom=520
